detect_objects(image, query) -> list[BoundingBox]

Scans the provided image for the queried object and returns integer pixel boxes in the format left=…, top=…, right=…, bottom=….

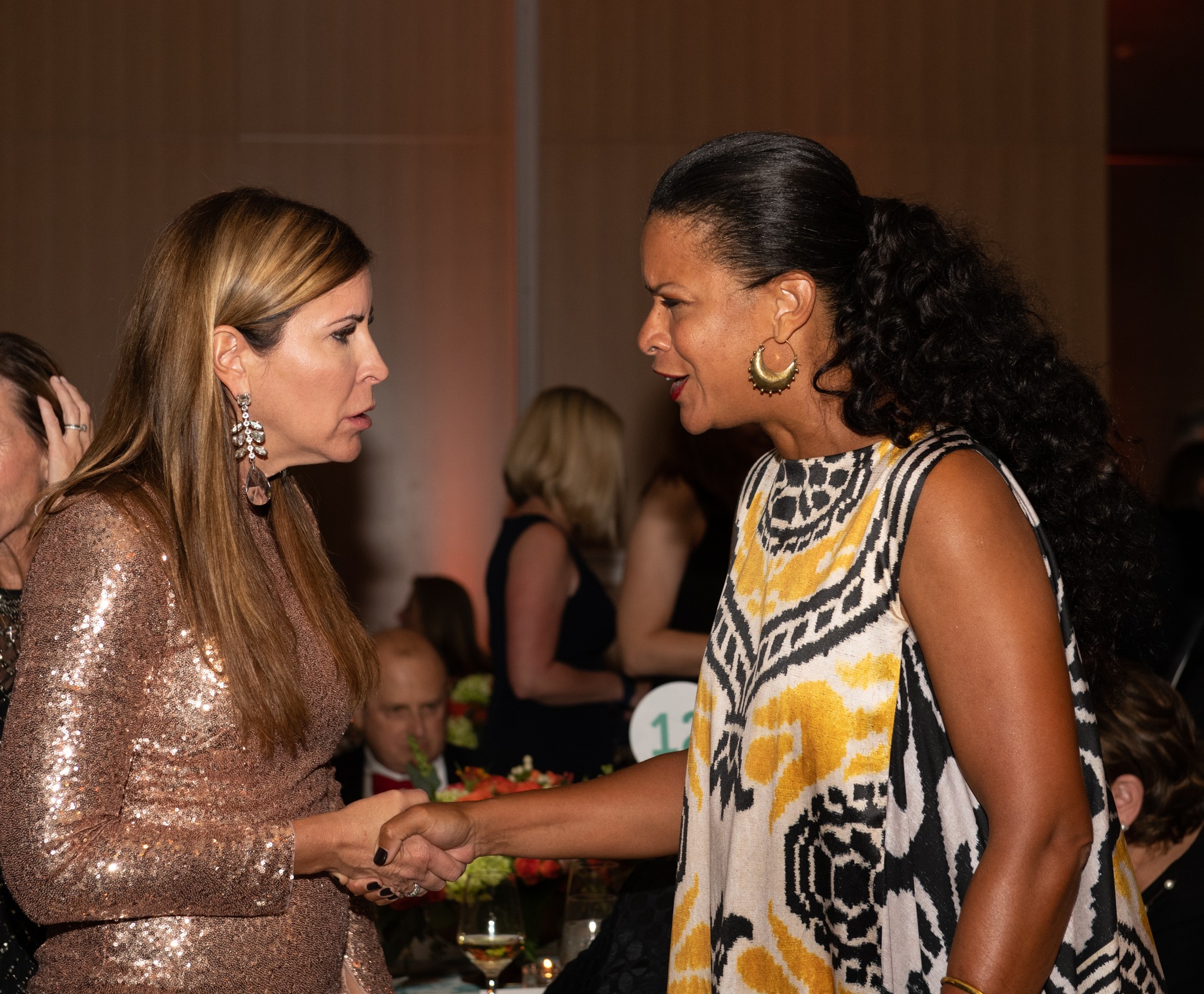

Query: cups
left=560, top=857, right=612, bottom=965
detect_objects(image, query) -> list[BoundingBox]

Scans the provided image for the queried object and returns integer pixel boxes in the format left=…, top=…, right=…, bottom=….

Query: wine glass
left=455, top=905, right=525, bottom=994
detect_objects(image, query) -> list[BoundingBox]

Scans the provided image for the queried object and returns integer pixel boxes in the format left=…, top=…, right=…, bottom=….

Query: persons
left=1, top=332, right=97, bottom=990
left=3, top=185, right=438, bottom=993
left=395, top=571, right=485, bottom=683
left=612, top=401, right=763, bottom=712
left=481, top=382, right=638, bottom=782
left=331, top=627, right=477, bottom=972
left=345, top=129, right=1170, bottom=994
left=1076, top=648, right=1204, bottom=993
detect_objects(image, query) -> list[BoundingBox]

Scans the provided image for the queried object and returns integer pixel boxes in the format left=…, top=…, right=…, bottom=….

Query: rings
left=403, top=883, right=420, bottom=898
left=63, top=423, right=82, bottom=431
